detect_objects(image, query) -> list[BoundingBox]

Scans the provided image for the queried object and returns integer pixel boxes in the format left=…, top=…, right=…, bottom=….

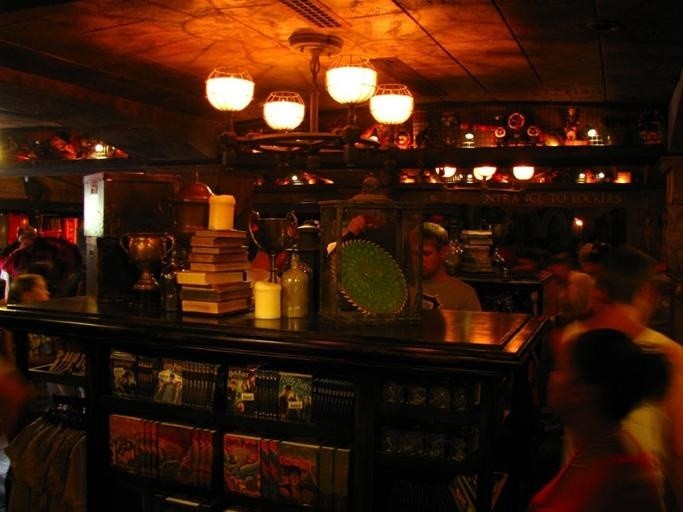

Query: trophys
left=244, top=209, right=306, bottom=282
left=119, top=229, right=177, bottom=316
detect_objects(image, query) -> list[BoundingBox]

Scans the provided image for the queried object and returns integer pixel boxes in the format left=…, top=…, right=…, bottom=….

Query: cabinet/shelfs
left=215, top=93, right=659, bottom=313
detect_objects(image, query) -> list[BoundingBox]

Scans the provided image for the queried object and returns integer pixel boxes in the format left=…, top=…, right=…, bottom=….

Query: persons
left=532, top=328, right=663, bottom=512
left=406, top=224, right=480, bottom=311
left=554, top=246, right=681, bottom=504
left=7, top=273, right=59, bottom=380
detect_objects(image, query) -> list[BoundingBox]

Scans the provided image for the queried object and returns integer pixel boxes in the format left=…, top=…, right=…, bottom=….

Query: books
left=176, top=228, right=249, bottom=325
left=223, top=433, right=352, bottom=507
left=459, top=229, right=494, bottom=272
left=105, top=416, right=214, bottom=490
left=104, top=353, right=218, bottom=409
left=225, top=367, right=364, bottom=425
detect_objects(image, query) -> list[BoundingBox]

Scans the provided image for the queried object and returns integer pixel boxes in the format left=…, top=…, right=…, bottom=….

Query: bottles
left=491, top=248, right=503, bottom=275
left=280, top=254, right=309, bottom=318
left=160, top=255, right=181, bottom=313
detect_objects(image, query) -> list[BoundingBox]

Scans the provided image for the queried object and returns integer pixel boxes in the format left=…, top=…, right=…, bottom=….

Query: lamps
left=202, top=56, right=258, bottom=112
left=371, top=80, right=414, bottom=126
left=327, top=54, right=377, bottom=105
left=262, top=88, right=306, bottom=134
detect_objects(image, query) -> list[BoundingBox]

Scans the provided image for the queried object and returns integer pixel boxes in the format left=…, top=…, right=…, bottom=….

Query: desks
left=0, top=293, right=556, bottom=508
